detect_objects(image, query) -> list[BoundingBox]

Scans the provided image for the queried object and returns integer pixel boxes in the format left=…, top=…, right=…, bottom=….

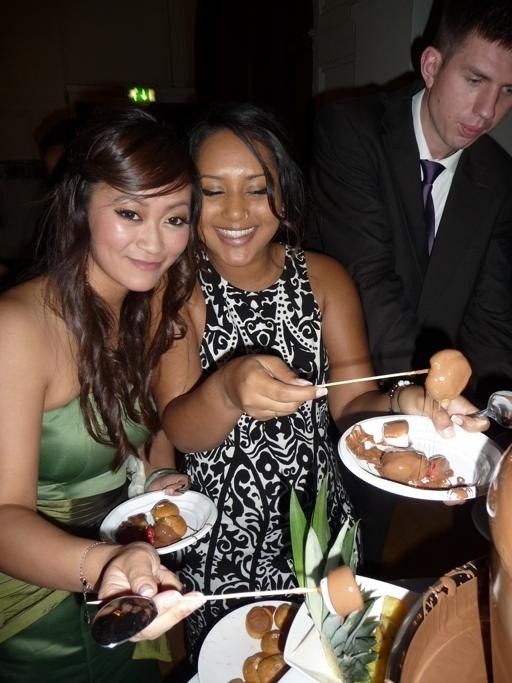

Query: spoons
left=91, top=594, right=159, bottom=648
left=464, top=390, right=512, bottom=429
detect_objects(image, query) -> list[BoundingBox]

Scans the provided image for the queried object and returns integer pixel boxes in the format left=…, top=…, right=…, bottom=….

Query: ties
left=419, top=158, right=446, bottom=256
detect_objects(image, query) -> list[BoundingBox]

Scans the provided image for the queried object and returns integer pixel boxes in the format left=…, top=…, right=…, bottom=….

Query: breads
left=115, top=500, right=187, bottom=548
left=229, top=602, right=299, bottom=683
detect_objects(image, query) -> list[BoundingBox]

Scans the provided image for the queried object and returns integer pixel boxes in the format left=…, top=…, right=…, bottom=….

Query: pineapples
left=288, top=475, right=408, bottom=683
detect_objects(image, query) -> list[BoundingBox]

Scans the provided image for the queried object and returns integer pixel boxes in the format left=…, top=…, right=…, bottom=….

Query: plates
left=97, top=487, right=219, bottom=556
left=337, top=412, right=505, bottom=503
left=195, top=598, right=320, bottom=683
left=282, top=568, right=423, bottom=683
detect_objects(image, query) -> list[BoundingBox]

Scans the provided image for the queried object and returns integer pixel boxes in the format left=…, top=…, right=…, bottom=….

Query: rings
left=273, top=409, right=279, bottom=420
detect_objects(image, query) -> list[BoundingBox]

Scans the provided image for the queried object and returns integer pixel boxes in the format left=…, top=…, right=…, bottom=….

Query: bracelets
left=78, top=539, right=111, bottom=596
left=386, top=379, right=417, bottom=412
left=143, top=468, right=180, bottom=492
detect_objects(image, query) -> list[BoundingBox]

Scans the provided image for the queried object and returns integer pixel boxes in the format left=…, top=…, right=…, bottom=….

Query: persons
left=291, top=0, right=512, bottom=592
left=0, top=98, right=208, bottom=683
left=147, top=100, right=496, bottom=677
left=37, top=120, right=89, bottom=181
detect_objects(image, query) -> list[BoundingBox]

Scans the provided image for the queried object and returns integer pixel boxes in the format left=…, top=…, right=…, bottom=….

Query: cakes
left=346, top=417, right=455, bottom=490
left=486, top=443, right=512, bottom=683
left=424, top=348, right=474, bottom=405
left=320, top=565, right=367, bottom=616
